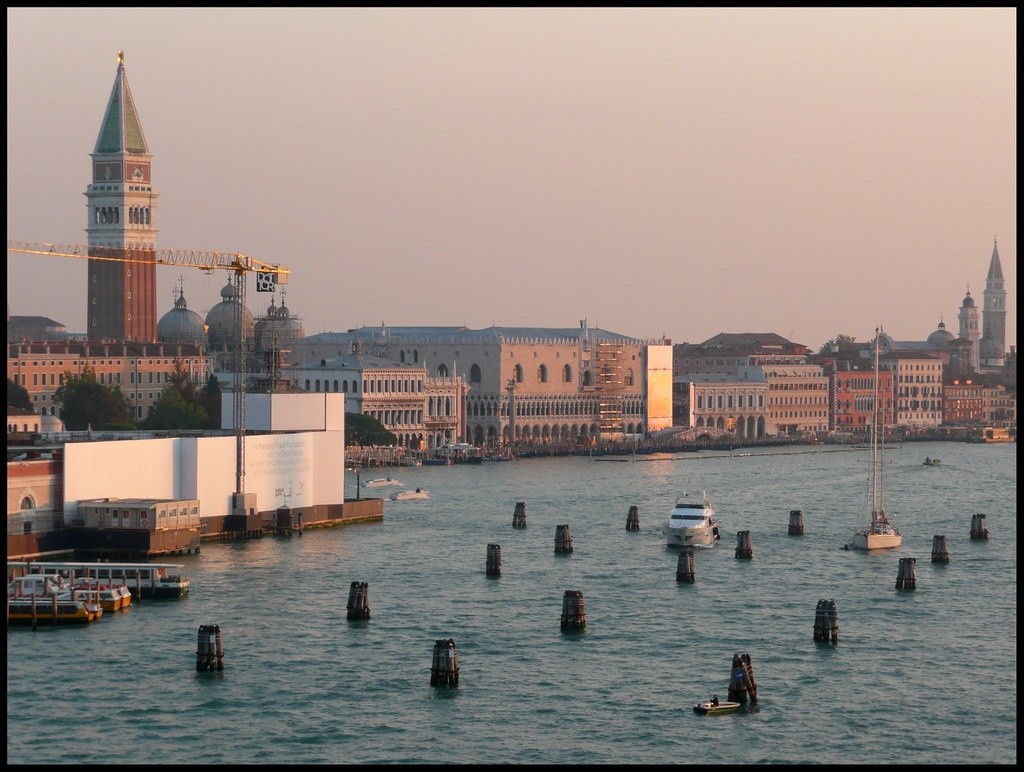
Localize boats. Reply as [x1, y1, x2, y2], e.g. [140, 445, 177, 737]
[359, 476, 402, 488]
[434, 442, 482, 464]
[423, 459, 451, 466]
[663, 480, 720, 551]
[922, 456, 941, 467]
[7, 561, 190, 622]
[389, 487, 431, 501]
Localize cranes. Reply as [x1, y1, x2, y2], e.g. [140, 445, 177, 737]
[7, 239, 290, 493]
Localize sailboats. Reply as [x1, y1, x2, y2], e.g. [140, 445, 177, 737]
[854, 327, 902, 549]
